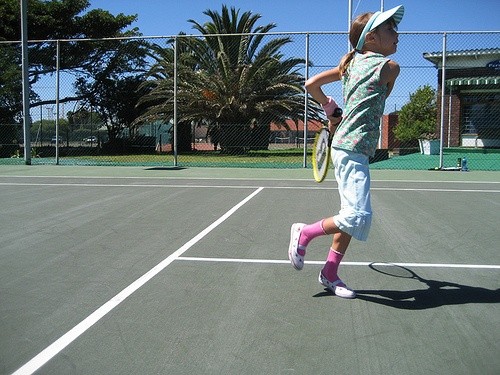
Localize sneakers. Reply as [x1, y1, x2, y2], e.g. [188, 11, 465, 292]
[288, 223, 304, 272]
[318, 271, 355, 299]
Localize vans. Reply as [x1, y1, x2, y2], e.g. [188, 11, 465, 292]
[52, 135, 63, 144]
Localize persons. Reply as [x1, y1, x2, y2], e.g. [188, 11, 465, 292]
[288, 4, 406, 299]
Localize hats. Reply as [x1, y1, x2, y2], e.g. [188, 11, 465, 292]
[356, 4, 405, 52]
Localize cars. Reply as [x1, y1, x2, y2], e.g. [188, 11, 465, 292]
[85, 136, 98, 143]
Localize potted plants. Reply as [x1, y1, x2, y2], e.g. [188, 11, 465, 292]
[391, 84, 440, 155]
